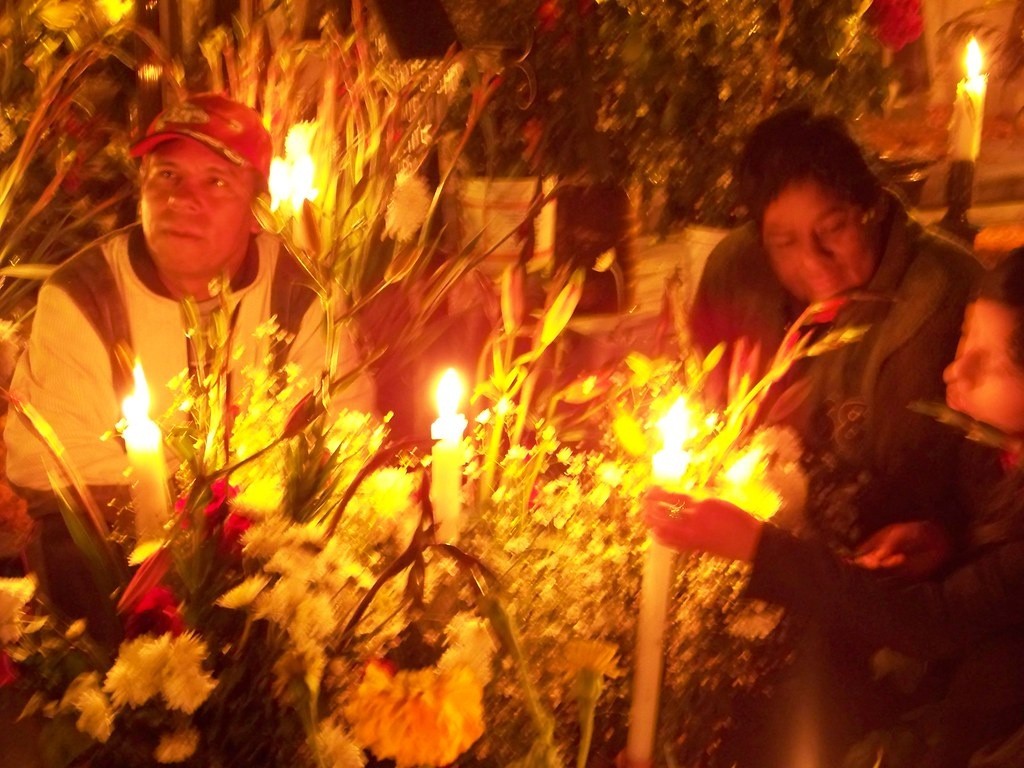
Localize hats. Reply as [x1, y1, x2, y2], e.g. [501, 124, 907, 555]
[127, 95, 272, 184]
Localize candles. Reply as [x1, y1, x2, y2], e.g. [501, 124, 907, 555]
[625, 397, 690, 768]
[119, 354, 169, 543]
[428, 369, 467, 548]
[946, 35, 988, 159]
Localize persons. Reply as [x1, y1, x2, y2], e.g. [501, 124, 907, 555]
[641, 246, 1024, 768]
[6, 92, 376, 521]
[686, 103, 1007, 560]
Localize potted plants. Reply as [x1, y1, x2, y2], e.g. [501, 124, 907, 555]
[597, 0, 887, 302]
[446, 45, 556, 276]
